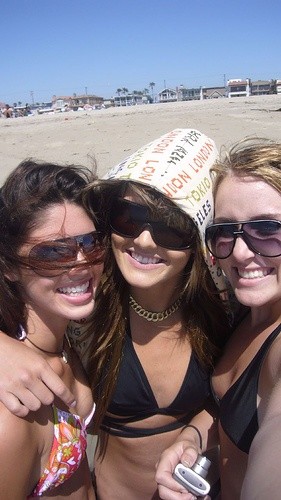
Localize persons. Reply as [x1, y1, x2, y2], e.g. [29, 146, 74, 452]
[0, 128, 230, 500]
[155, 137, 281, 500]
[0, 159, 105, 500]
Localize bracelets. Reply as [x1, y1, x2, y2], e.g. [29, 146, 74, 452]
[181, 424, 202, 449]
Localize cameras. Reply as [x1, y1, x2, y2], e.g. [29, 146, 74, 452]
[172, 454, 213, 497]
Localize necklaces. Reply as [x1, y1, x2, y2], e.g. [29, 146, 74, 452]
[26, 337, 68, 363]
[129, 295, 181, 322]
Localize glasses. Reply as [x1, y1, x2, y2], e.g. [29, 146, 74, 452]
[27, 230, 108, 279]
[203, 219, 280, 259]
[106, 198, 199, 252]
